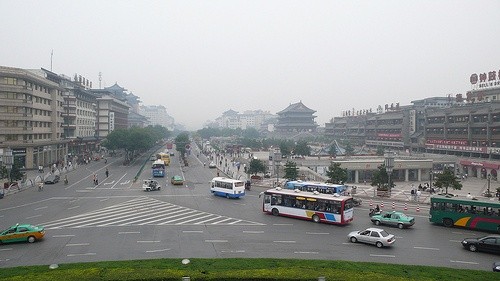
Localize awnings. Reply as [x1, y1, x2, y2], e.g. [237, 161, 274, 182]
[459, 161, 500, 169]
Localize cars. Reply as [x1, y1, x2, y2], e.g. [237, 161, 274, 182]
[43, 174, 60, 184]
[492, 262, 500, 272]
[348, 228, 396, 248]
[170, 175, 184, 185]
[209, 161, 216, 168]
[461, 235, 500, 256]
[371, 211, 415, 229]
[170, 152, 174, 156]
[165, 149, 169, 153]
[0, 223, 46, 246]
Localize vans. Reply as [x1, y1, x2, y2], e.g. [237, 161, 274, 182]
[151, 155, 156, 161]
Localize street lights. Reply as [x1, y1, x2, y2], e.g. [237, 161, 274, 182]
[58, 140, 73, 164]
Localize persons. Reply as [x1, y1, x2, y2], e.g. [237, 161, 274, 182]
[463, 173, 467, 180]
[458, 206, 500, 216]
[375, 204, 380, 212]
[220, 161, 222, 166]
[295, 187, 335, 194]
[272, 197, 341, 214]
[217, 169, 220, 176]
[466, 192, 472, 199]
[94, 175, 98, 185]
[418, 183, 443, 193]
[106, 169, 109, 177]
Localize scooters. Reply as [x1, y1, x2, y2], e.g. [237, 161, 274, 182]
[369, 208, 382, 216]
[245, 184, 251, 191]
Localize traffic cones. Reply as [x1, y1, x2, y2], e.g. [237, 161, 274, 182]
[416, 204, 421, 212]
[392, 202, 396, 209]
[369, 199, 373, 206]
[404, 203, 408, 210]
[380, 200, 384, 208]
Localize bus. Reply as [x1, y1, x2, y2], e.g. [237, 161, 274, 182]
[284, 180, 349, 196]
[208, 177, 245, 199]
[156, 152, 170, 166]
[151, 159, 165, 177]
[428, 197, 500, 235]
[167, 143, 172, 149]
[259, 187, 353, 225]
